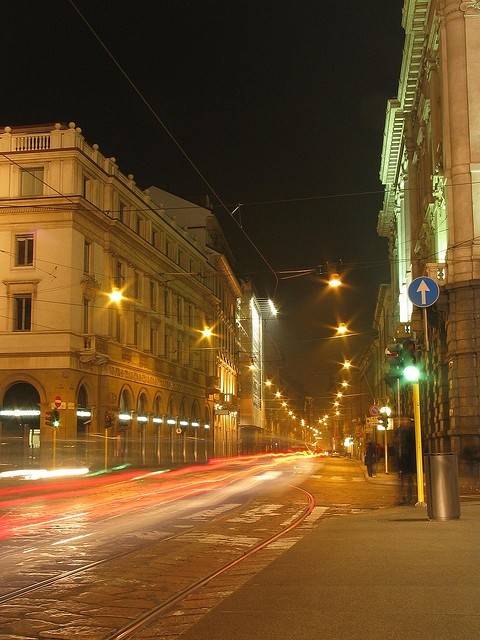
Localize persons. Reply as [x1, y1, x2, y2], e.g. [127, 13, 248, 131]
[364, 442, 376, 478]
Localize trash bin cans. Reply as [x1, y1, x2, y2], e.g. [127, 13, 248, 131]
[424, 452, 461, 522]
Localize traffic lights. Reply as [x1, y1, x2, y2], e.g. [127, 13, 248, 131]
[386, 340, 420, 382]
[45, 410, 60, 426]
[104, 412, 114, 427]
[378, 413, 388, 428]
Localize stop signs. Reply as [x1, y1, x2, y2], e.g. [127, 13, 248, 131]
[369, 404, 381, 417]
[54, 396, 61, 407]
